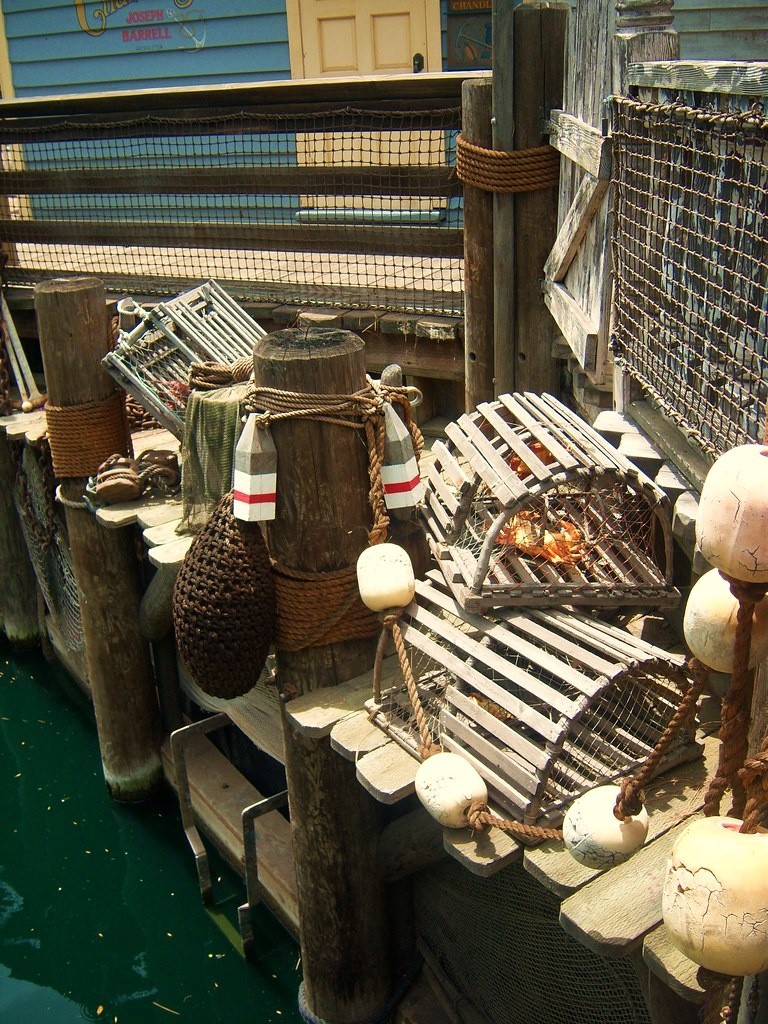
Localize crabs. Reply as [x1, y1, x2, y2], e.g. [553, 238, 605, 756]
[481, 441, 587, 564]
